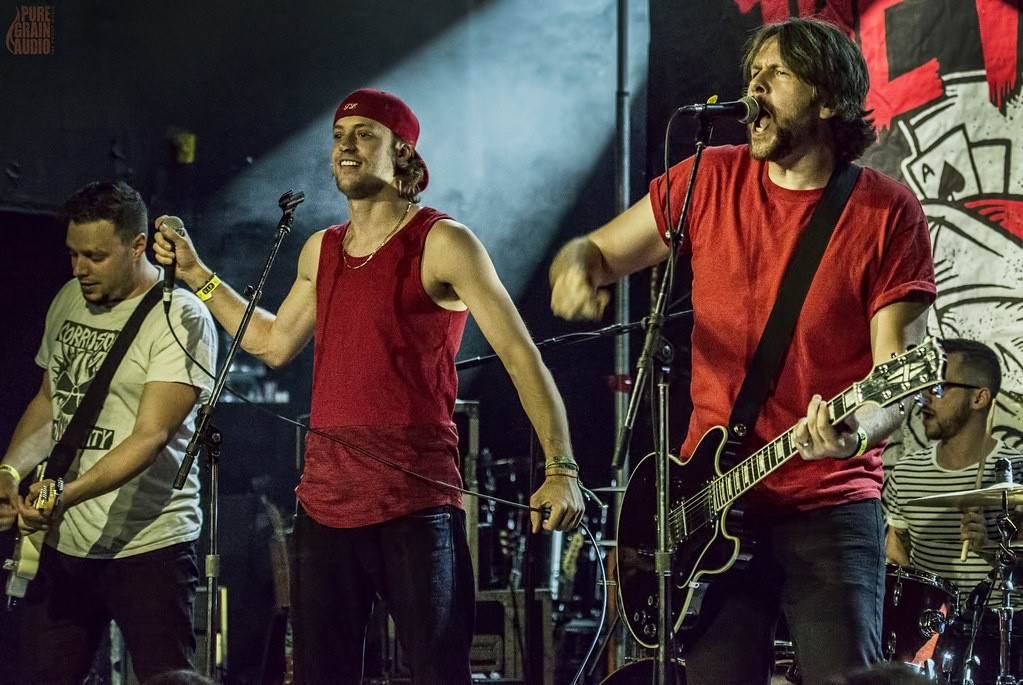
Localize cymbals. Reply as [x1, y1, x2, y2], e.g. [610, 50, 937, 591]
[907, 480, 1023, 507]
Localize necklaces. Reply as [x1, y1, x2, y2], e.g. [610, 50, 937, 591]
[339, 196, 411, 271]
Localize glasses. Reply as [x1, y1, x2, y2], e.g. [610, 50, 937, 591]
[942, 381, 981, 394]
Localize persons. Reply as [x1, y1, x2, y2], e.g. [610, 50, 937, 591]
[548, 19, 937, 685]
[149, 90, 585, 685]
[0, 182, 219, 685]
[884, 339, 1023, 647]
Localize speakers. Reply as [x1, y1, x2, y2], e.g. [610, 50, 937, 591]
[120, 585, 227, 685]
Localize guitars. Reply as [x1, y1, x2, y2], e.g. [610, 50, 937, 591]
[4, 461, 63, 598]
[614, 333, 949, 652]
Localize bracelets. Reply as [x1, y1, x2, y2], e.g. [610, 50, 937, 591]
[0, 464, 20, 484]
[834, 426, 868, 460]
[192, 273, 223, 301]
[544, 455, 578, 478]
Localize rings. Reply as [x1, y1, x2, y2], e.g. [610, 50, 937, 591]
[799, 435, 811, 449]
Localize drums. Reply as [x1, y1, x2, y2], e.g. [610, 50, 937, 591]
[597, 656, 688, 685]
[881, 563, 958, 677]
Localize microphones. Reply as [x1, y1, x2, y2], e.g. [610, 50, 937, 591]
[161, 216, 184, 313]
[678, 95, 761, 124]
[966, 568, 995, 611]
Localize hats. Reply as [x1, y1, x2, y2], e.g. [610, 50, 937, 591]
[333, 89, 420, 146]
